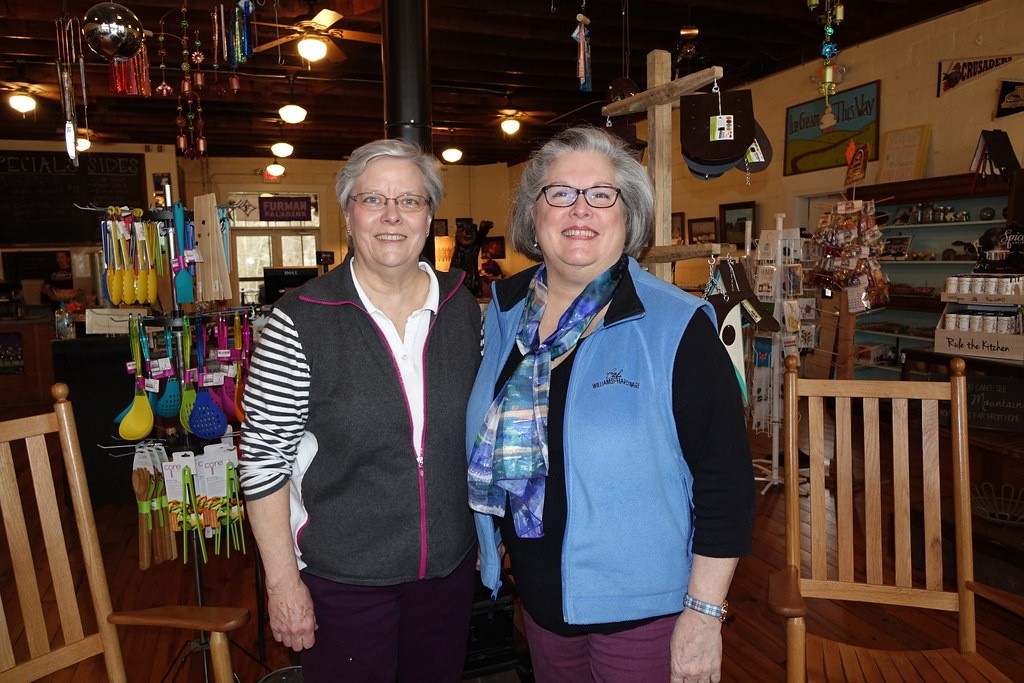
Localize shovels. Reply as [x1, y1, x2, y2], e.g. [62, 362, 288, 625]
[174, 201, 194, 304]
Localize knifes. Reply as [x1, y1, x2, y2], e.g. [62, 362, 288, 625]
[63, 71, 75, 159]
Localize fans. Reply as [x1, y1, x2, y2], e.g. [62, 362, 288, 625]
[77, 124, 126, 142]
[0, 62, 97, 106]
[250, 0, 382, 63]
[483, 91, 557, 127]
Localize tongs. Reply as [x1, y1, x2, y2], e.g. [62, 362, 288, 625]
[215, 526, 221, 555]
[230, 523, 240, 550]
[115, 206, 136, 305]
[182, 467, 209, 564]
[103, 209, 111, 302]
[107, 206, 124, 305]
[134, 208, 157, 304]
[226, 462, 246, 558]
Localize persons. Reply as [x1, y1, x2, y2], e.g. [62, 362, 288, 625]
[465, 129, 756, 683]
[236, 140, 482, 683]
[45, 251, 83, 314]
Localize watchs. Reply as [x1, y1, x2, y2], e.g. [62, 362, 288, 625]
[683, 594, 728, 623]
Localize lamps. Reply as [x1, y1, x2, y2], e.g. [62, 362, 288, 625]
[271, 120, 294, 157]
[500, 92, 522, 136]
[278, 76, 308, 124]
[75, 124, 91, 152]
[297, 0, 327, 62]
[266, 155, 285, 176]
[8, 62, 38, 113]
[442, 128, 463, 163]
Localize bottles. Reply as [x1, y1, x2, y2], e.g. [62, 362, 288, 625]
[0, 344, 24, 375]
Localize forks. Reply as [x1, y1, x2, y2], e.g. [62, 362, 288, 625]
[132, 465, 177, 570]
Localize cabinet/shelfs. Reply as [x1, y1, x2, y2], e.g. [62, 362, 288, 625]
[834, 169, 1024, 421]
[825, 410, 1024, 618]
[934, 273, 1024, 365]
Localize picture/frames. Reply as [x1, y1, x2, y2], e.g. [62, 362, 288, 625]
[783, 80, 881, 177]
[434, 219, 449, 236]
[719, 201, 757, 250]
[688, 217, 719, 258]
[672, 212, 685, 245]
[873, 123, 932, 185]
[481, 236, 506, 259]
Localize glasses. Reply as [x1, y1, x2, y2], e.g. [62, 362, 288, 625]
[348, 192, 430, 212]
[536, 185, 630, 212]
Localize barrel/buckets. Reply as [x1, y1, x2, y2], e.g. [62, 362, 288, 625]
[21, 280, 45, 306]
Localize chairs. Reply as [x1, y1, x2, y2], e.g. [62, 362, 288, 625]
[767, 356, 1024, 683]
[0, 383, 251, 683]
